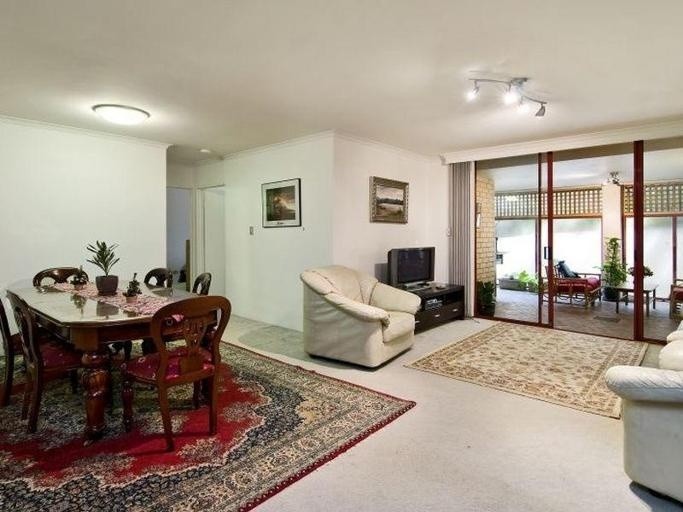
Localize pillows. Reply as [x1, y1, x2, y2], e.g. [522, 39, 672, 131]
[558, 260, 580, 279]
[659, 339, 683, 371]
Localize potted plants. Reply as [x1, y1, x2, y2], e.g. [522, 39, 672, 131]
[97, 301, 120, 320]
[70, 265, 88, 290]
[70, 293, 89, 314]
[86, 240, 120, 294]
[527, 272, 538, 292]
[518, 270, 530, 291]
[121, 273, 142, 303]
[591, 236, 629, 300]
[477, 280, 497, 317]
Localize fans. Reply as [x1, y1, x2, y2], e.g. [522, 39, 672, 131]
[602, 171, 623, 188]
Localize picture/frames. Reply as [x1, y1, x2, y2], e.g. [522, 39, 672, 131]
[544, 246, 547, 259]
[369, 176, 410, 224]
[262, 178, 303, 228]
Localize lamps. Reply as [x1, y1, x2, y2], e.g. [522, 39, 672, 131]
[91, 104, 151, 125]
[467, 77, 547, 116]
[475, 203, 481, 229]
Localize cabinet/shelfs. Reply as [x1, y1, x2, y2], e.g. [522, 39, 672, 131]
[394, 282, 465, 335]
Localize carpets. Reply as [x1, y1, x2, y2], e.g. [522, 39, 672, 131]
[402, 322, 650, 420]
[0, 338, 417, 512]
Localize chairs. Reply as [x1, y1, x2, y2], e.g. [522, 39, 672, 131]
[141, 272, 211, 356]
[541, 264, 601, 310]
[33, 266, 89, 288]
[123, 268, 172, 356]
[668, 279, 683, 319]
[1, 299, 49, 405]
[6, 290, 114, 433]
[118, 295, 231, 451]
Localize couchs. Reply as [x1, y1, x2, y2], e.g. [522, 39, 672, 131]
[300, 265, 420, 372]
[604, 329, 683, 504]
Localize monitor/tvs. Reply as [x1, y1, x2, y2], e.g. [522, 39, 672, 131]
[387, 246, 435, 287]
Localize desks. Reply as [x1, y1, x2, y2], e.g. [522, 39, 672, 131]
[5, 282, 217, 439]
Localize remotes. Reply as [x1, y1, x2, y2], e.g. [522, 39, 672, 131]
[436, 284, 446, 289]
